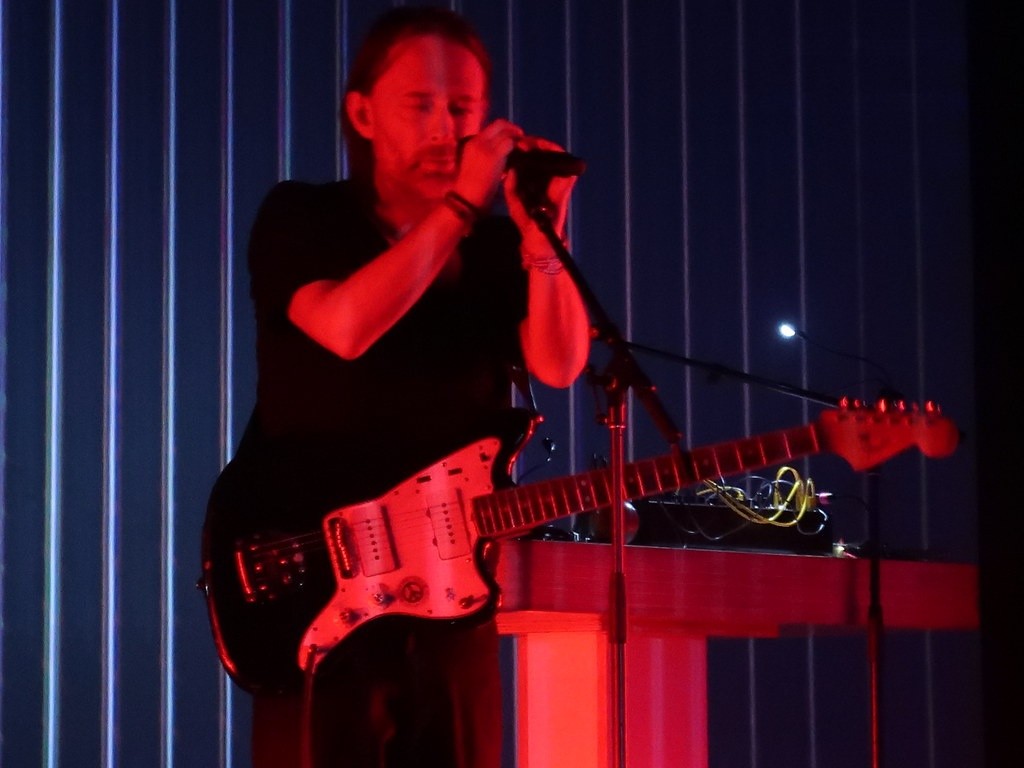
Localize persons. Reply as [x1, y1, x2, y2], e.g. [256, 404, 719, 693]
[248, 3, 590, 768]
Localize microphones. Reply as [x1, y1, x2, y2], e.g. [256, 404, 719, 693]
[455, 135, 586, 179]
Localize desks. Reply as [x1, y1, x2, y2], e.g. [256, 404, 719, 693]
[483, 539, 979, 768]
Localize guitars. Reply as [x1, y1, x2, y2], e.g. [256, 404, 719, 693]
[202, 394, 960, 684]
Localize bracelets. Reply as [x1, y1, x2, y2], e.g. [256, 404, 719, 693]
[534, 260, 564, 275]
